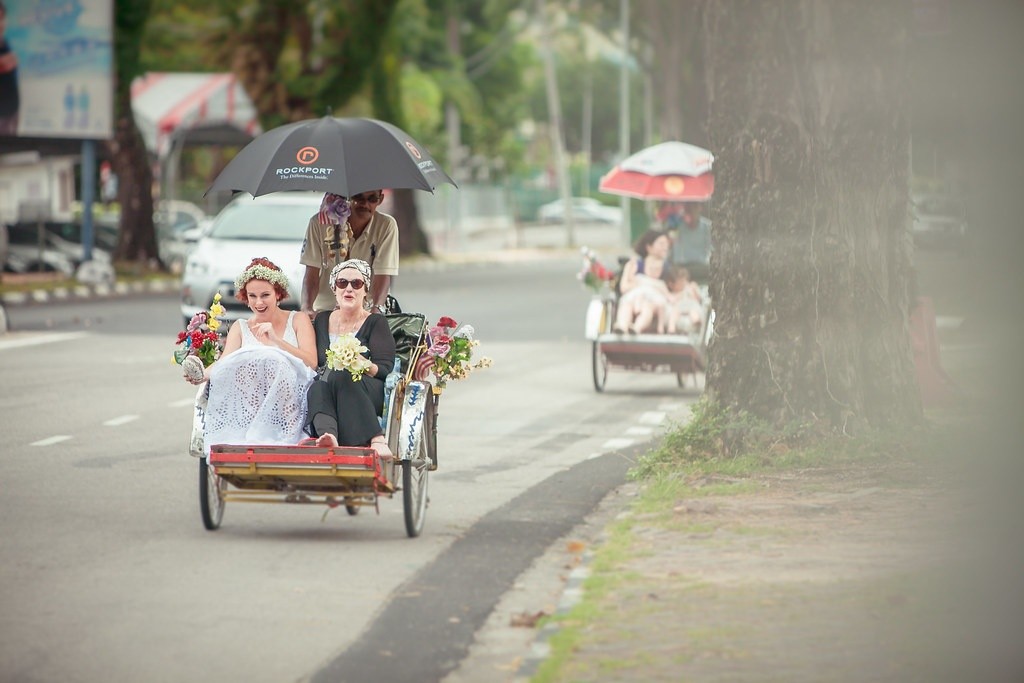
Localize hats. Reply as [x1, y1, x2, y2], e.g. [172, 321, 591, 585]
[328, 258, 371, 294]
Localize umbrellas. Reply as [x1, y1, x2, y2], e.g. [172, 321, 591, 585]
[202, 105, 459, 200]
[599, 141, 714, 202]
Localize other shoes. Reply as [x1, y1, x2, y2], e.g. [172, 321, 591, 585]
[371, 438, 393, 460]
[315, 432, 339, 447]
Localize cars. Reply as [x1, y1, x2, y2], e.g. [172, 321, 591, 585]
[173, 189, 333, 332]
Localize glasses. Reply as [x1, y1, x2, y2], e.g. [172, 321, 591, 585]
[335, 278, 364, 290]
[350, 194, 381, 203]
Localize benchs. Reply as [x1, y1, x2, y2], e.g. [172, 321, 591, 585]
[617, 258, 710, 297]
[311, 312, 430, 381]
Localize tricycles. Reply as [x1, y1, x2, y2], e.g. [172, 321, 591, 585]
[587, 239, 714, 392]
[187, 300, 440, 538]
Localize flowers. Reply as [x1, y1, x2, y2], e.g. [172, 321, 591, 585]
[423, 315, 492, 387]
[578, 250, 615, 296]
[320, 333, 373, 383]
[171, 292, 226, 367]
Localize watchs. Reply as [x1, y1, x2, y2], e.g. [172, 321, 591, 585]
[373, 304, 385, 313]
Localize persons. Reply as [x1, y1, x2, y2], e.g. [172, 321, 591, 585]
[182, 256, 318, 464]
[298, 189, 400, 320]
[302, 259, 396, 459]
[670, 201, 712, 264]
[613, 230, 702, 336]
